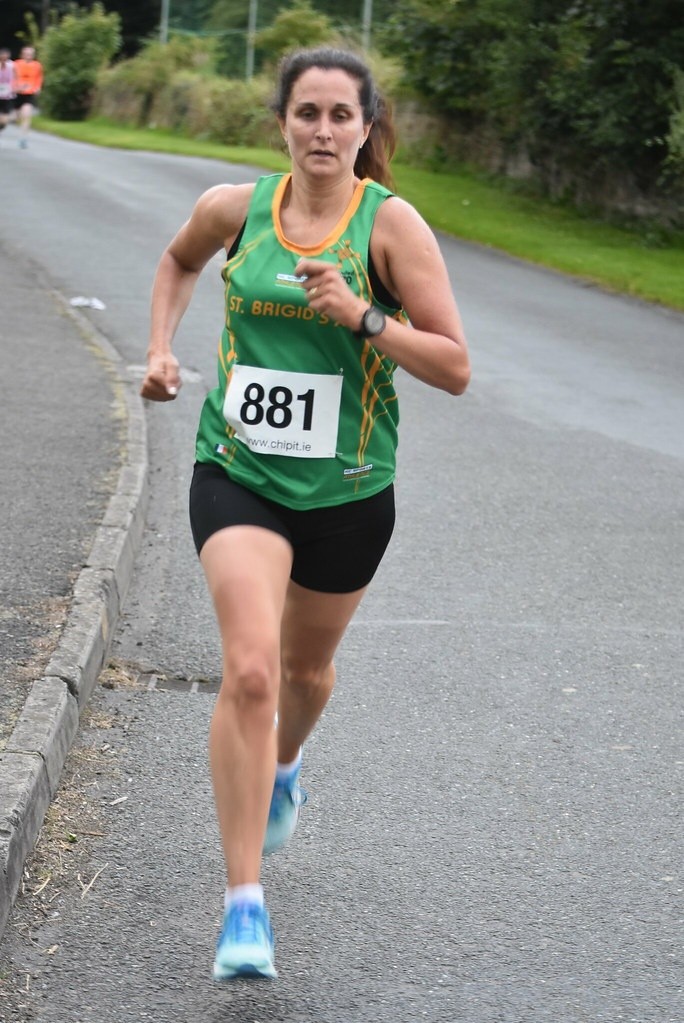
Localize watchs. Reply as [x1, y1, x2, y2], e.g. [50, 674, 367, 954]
[352, 304, 386, 341]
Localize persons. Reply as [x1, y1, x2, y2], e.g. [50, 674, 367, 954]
[0, 49, 17, 131]
[139, 51, 469, 984]
[11, 46, 44, 149]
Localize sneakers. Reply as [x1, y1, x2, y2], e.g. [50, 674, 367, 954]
[211, 903, 277, 979]
[263, 764, 302, 856]
